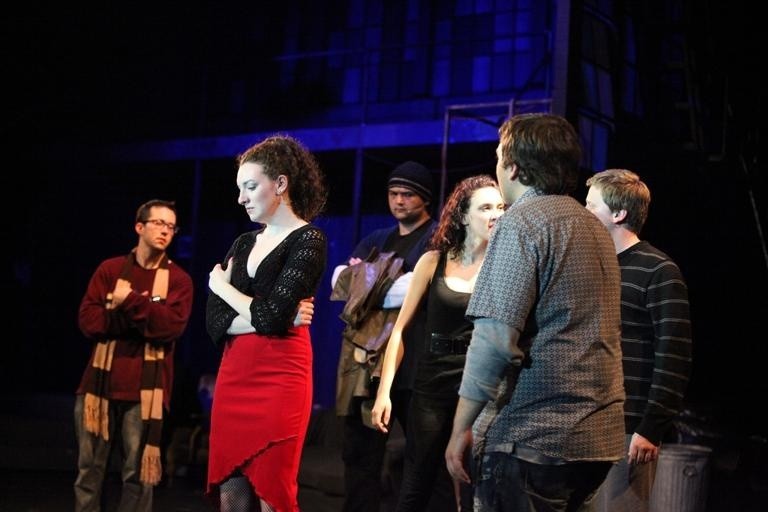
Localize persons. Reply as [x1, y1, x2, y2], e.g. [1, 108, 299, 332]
[70, 199, 195, 511]
[366, 174, 506, 511]
[442, 112, 629, 511]
[200, 132, 329, 511]
[580, 167, 721, 511]
[328, 160, 444, 512]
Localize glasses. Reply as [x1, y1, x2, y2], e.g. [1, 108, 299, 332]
[144, 219, 179, 233]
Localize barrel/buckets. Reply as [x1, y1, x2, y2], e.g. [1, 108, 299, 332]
[648, 444, 712, 512]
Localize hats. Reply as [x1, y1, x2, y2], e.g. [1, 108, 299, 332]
[388, 161, 432, 202]
